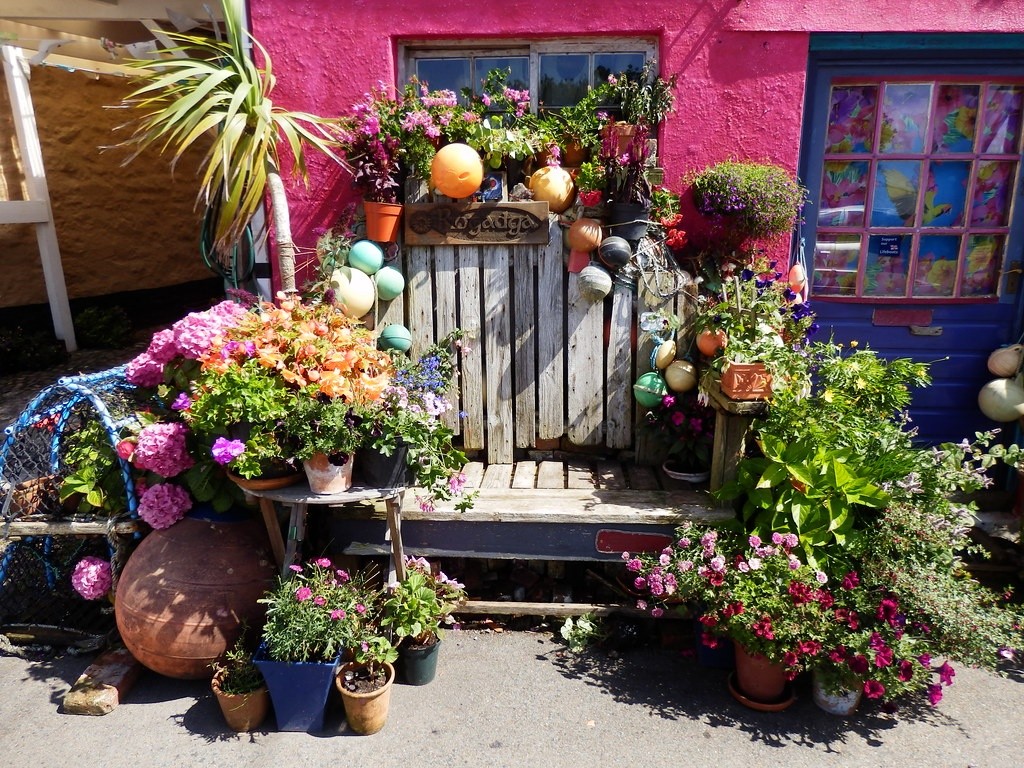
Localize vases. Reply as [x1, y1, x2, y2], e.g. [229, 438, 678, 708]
[362, 201, 401, 244]
[212, 667, 269, 731]
[393, 632, 441, 686]
[335, 659, 396, 735]
[253, 641, 343, 731]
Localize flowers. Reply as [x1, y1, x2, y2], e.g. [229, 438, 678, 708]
[550, 152, 1023, 716]
[327, 76, 669, 205]
[34, 286, 474, 667]
